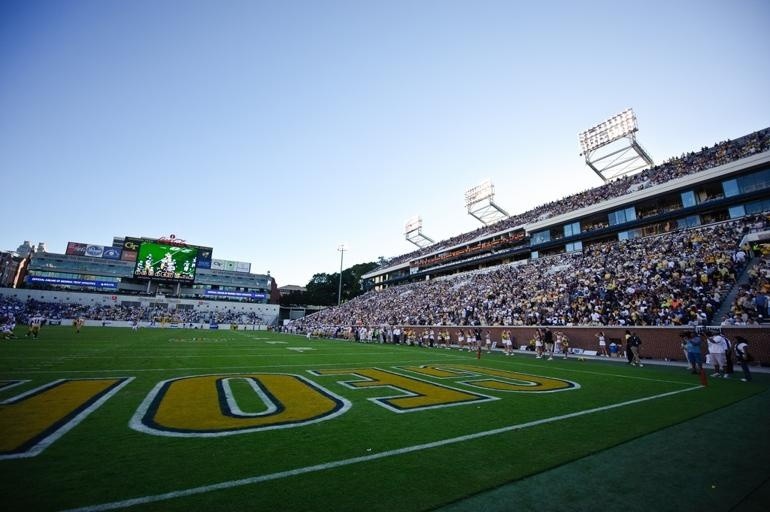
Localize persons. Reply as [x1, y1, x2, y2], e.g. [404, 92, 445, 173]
[2, 281, 271, 340]
[271, 128, 770, 380]
[136, 252, 197, 279]
